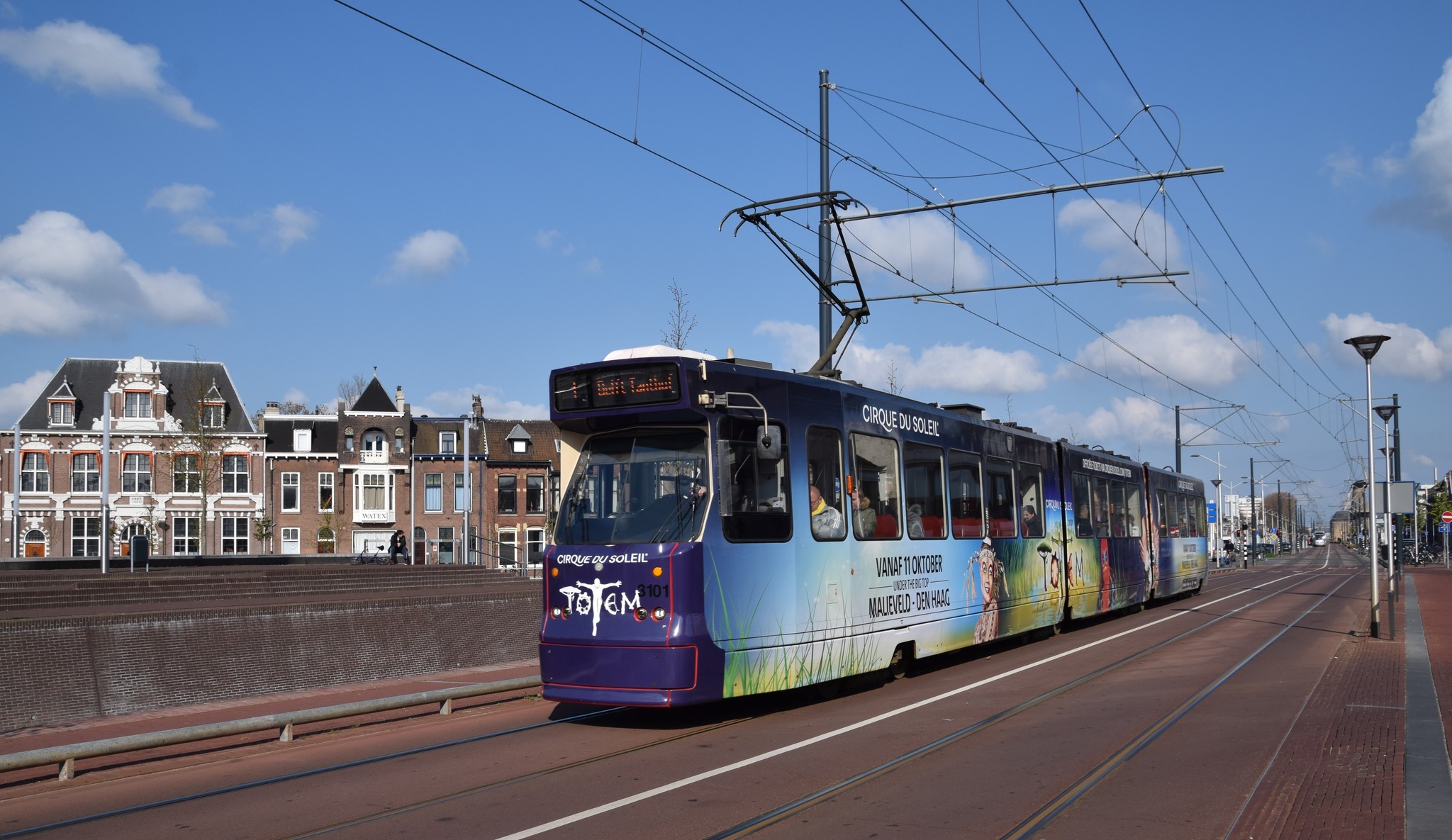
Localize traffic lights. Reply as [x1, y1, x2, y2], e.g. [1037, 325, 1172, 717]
[1235, 529, 1241, 539]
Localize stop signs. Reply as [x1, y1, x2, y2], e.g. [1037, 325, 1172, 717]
[1441, 511, 1452, 523]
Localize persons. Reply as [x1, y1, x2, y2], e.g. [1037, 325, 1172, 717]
[810, 485, 844, 538]
[1023, 506, 1043, 536]
[1075, 488, 1140, 537]
[852, 485, 877, 538]
[690, 464, 744, 512]
[1223, 539, 1235, 561]
[1310, 536, 1313, 546]
[1159, 508, 1196, 536]
[389, 529, 410, 565]
[894, 500, 924, 537]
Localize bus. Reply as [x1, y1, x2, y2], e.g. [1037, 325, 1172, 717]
[539, 190, 1208, 710]
[1313, 523, 1327, 546]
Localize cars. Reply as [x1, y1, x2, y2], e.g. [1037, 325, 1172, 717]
[1212, 546, 1236, 562]
[1246, 542, 1291, 558]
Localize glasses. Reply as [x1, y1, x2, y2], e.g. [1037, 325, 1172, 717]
[811, 497, 820, 506]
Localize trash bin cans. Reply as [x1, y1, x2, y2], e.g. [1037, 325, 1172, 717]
[129, 537, 148, 564]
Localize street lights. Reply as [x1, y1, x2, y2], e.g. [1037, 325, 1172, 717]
[1209, 479, 1224, 569]
[1342, 334, 1393, 639]
[1371, 405, 1402, 591]
[1241, 475, 1269, 560]
[1238, 495, 1259, 558]
[1245, 500, 1289, 544]
[1221, 481, 1250, 545]
[411, 437, 417, 566]
[1190, 451, 1227, 566]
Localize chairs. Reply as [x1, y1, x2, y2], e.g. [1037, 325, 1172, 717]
[977, 516, 1000, 537]
[939, 517, 965, 539]
[874, 514, 898, 537]
[1112, 523, 1123, 537]
[996, 519, 1015, 537]
[920, 516, 943, 538]
[960, 517, 983, 537]
[1011, 518, 1031, 536]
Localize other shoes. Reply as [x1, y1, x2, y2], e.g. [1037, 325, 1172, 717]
[1228, 558, 1231, 561]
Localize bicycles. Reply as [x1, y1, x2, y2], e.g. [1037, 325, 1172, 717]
[1402, 542, 1452, 566]
[1360, 545, 1381, 557]
[1221, 547, 1233, 568]
[350, 545, 390, 565]
